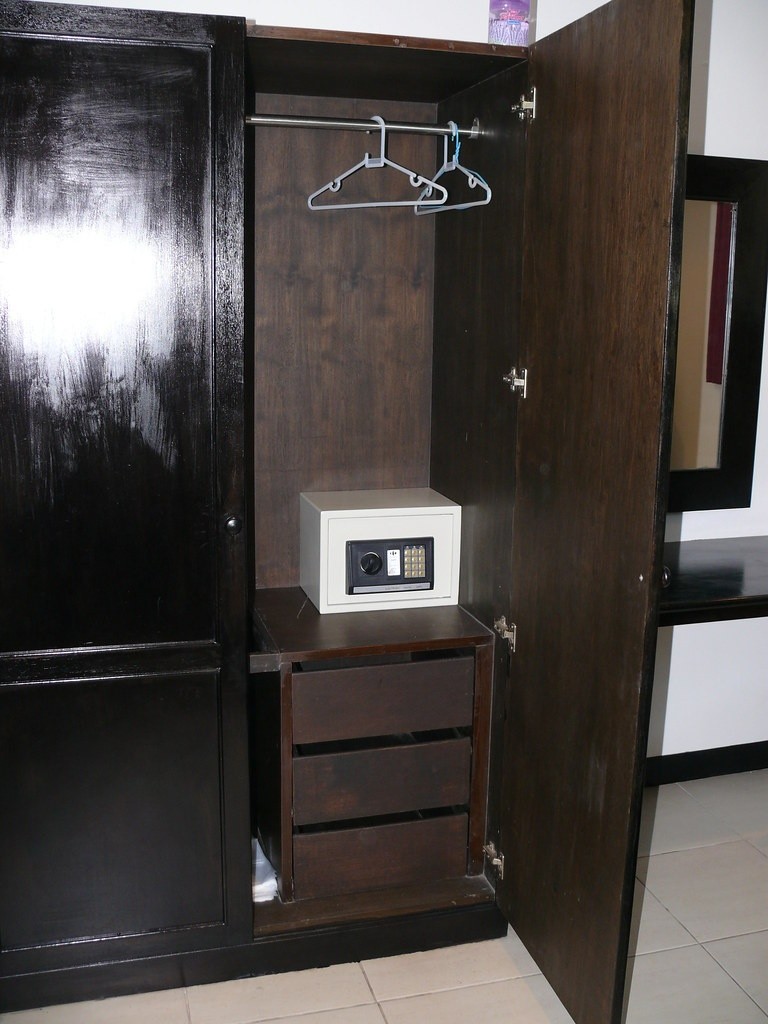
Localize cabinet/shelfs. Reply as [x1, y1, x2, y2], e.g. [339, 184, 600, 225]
[0, 0, 695, 1024]
[644, 535, 768, 787]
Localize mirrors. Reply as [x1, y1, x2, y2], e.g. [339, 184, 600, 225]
[666, 153, 768, 512]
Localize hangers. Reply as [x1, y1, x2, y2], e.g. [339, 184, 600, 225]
[307, 115, 446, 210]
[419, 124, 488, 208]
[414, 121, 491, 215]
